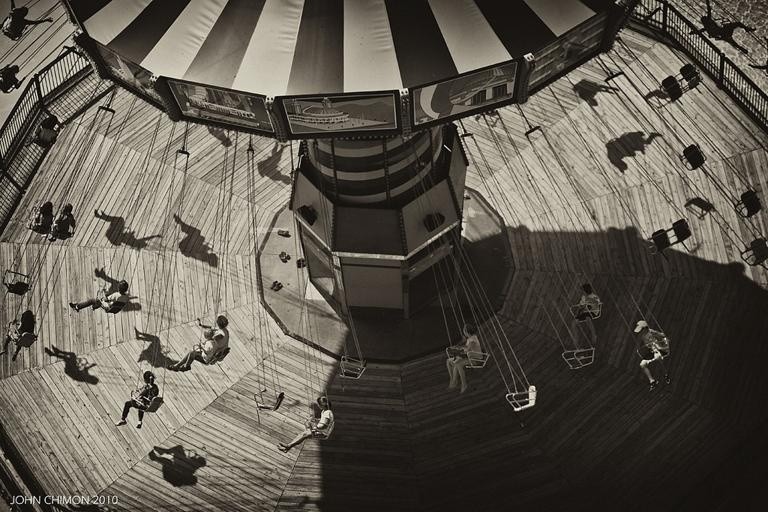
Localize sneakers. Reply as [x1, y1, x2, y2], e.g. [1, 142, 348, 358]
[69, 302, 80, 313]
[277, 441, 291, 453]
[116, 420, 126, 426]
[0, 350, 6, 356]
[12, 352, 17, 362]
[648, 380, 658, 392]
[136, 421, 142, 428]
[167, 364, 191, 372]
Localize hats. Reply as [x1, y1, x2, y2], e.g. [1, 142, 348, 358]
[634, 320, 648, 333]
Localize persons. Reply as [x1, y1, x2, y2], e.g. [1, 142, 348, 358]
[444, 323, 484, 395]
[571, 283, 600, 349]
[0, 0, 54, 41]
[114, 370, 158, 430]
[69, 280, 130, 314]
[634, 320, 672, 392]
[1, 63, 27, 92]
[25, 202, 77, 241]
[168, 314, 230, 372]
[0, 309, 35, 361]
[33, 113, 59, 148]
[277, 395, 335, 455]
